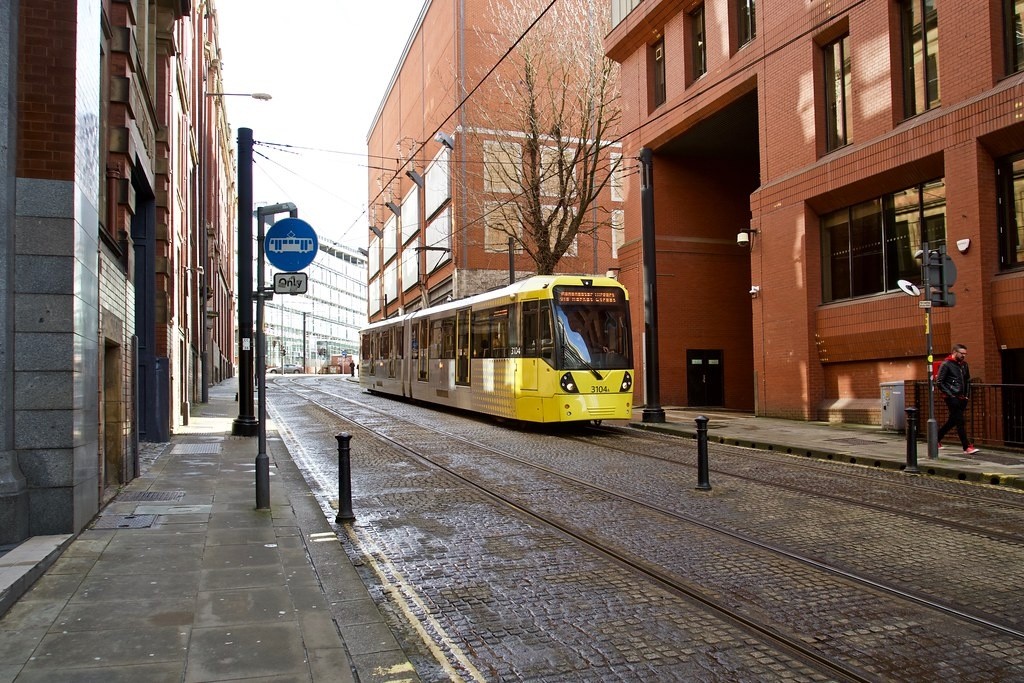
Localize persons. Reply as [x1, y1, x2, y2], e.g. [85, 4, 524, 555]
[349, 360, 355, 376]
[936, 345, 980, 455]
[570, 319, 608, 357]
[479, 331, 502, 358]
[356, 362, 359, 377]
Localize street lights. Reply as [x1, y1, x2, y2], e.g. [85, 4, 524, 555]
[202, 92, 274, 405]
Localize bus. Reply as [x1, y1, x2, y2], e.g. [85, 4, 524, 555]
[359, 274, 635, 435]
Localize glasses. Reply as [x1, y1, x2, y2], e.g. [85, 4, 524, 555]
[956, 350, 969, 355]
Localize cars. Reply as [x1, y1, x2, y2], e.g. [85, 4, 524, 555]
[266, 364, 303, 375]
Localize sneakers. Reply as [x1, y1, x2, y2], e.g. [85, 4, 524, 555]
[938, 442, 945, 450]
[963, 444, 980, 454]
